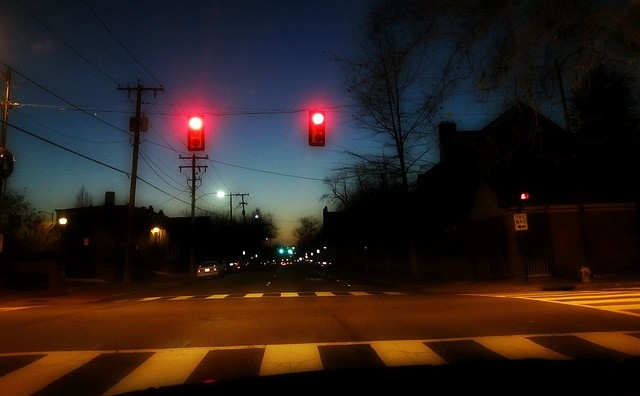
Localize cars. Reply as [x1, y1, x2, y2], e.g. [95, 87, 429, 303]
[196, 260, 226, 280]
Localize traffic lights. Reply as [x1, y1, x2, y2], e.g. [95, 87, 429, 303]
[187, 114, 205, 151]
[519, 190, 530, 202]
[309, 108, 325, 145]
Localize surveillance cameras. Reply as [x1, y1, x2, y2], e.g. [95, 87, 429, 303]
[519, 192, 531, 201]
